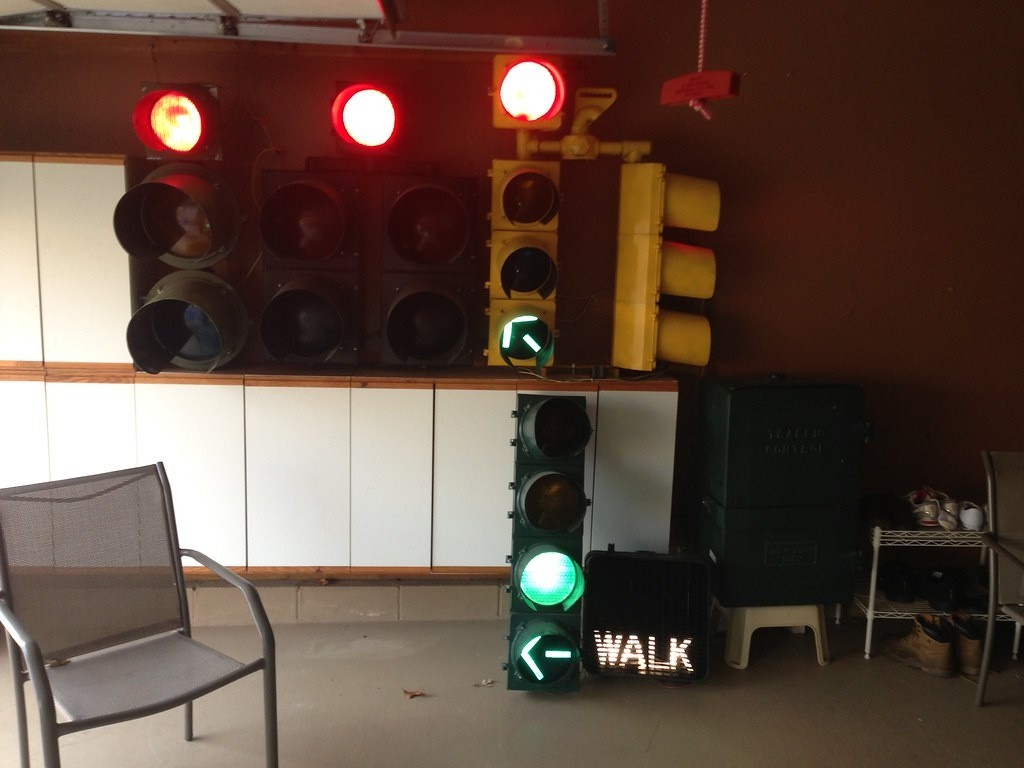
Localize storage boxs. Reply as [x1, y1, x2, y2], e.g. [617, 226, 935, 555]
[681, 475, 864, 609]
[688, 377, 874, 509]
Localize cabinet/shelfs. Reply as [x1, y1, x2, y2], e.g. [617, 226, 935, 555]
[1, 151, 682, 581]
[836, 509, 1022, 661]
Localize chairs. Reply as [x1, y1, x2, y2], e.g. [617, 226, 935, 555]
[0, 460, 279, 767]
[974, 448, 1024, 710]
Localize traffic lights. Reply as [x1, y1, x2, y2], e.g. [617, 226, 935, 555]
[478, 157, 559, 373]
[577, 543, 713, 686]
[610, 157, 722, 375]
[489, 50, 567, 133]
[501, 389, 594, 694]
[111, 76, 490, 372]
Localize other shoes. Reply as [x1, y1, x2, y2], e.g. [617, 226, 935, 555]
[889, 564, 960, 610]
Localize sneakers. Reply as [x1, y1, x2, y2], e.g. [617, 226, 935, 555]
[866, 486, 984, 532]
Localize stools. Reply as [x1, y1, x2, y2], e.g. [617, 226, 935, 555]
[710, 595, 833, 670]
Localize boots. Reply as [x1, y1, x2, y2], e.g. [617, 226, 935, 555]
[945, 612, 983, 674]
[876, 612, 955, 678]
